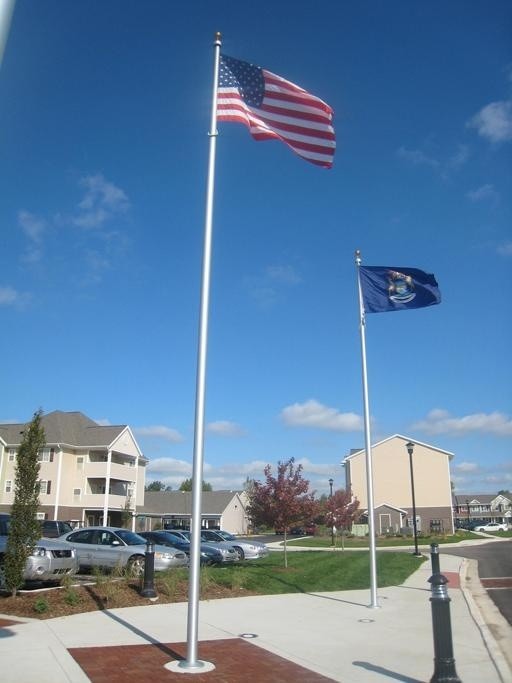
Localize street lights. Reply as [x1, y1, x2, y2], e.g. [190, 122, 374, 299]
[328, 478, 335, 546]
[405, 440, 422, 556]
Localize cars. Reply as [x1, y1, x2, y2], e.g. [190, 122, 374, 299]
[158, 530, 240, 562]
[135, 531, 224, 567]
[474, 522, 509, 532]
[212, 530, 270, 561]
[58, 525, 188, 578]
[0, 512, 80, 590]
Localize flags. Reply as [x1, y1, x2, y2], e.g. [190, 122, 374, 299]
[358, 264, 443, 315]
[213, 29, 336, 171]
